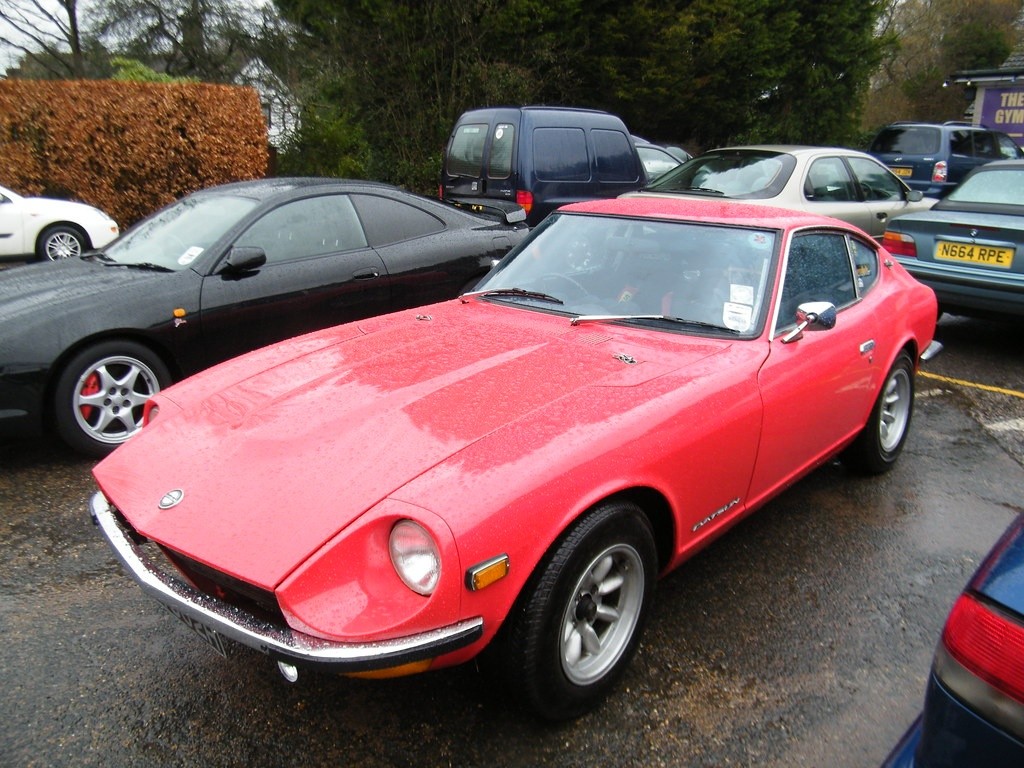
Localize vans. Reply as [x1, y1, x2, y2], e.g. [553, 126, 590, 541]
[438, 106, 684, 228]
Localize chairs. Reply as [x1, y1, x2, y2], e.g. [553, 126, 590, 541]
[663, 237, 762, 335]
[596, 234, 682, 320]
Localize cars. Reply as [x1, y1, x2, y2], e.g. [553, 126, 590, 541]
[882, 160, 1024, 322]
[879, 510, 1024, 768]
[90, 198, 942, 724]
[0, 184, 119, 262]
[0, 177, 530, 459]
[616, 145, 939, 244]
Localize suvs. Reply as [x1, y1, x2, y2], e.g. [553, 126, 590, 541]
[862, 121, 1024, 201]
